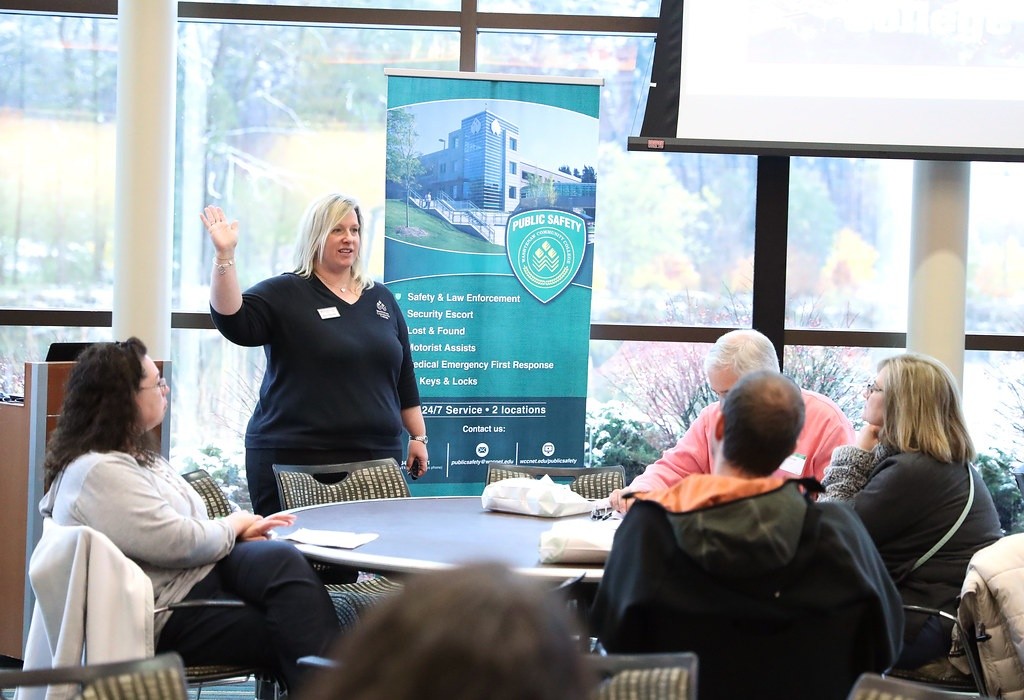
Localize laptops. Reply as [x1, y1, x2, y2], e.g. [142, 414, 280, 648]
[8, 341, 115, 403]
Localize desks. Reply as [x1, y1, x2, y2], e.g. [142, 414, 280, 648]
[240, 495, 639, 584]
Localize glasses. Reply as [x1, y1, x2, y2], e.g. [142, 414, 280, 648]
[865, 384, 882, 394]
[138, 378, 167, 390]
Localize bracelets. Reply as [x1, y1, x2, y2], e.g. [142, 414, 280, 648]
[212, 257, 236, 275]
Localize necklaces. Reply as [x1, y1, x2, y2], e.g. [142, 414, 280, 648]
[316, 271, 354, 292]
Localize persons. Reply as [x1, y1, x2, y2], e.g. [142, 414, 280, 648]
[200, 193, 431, 516]
[611, 330, 855, 515]
[36, 338, 351, 700]
[308, 560, 598, 700]
[811, 352, 1006, 666]
[590, 370, 907, 700]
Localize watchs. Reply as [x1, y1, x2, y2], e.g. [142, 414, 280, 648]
[408, 435, 429, 444]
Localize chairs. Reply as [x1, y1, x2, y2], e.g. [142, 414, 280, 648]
[278, 457, 429, 618]
[485, 455, 634, 508]
